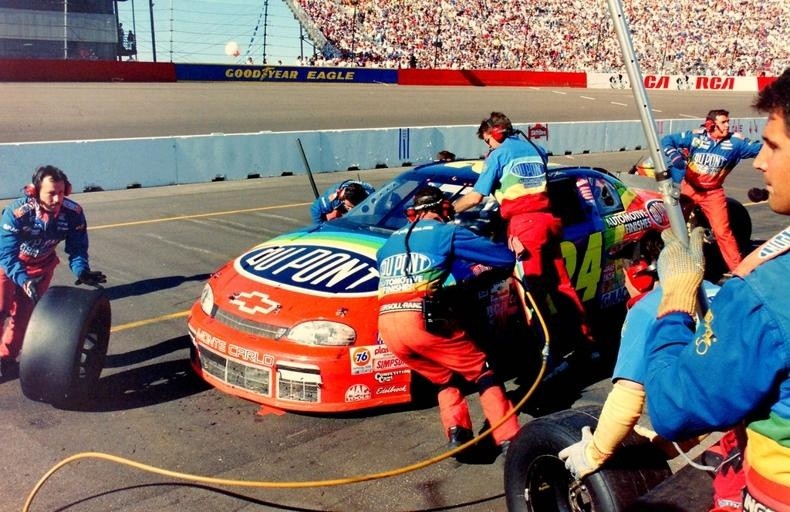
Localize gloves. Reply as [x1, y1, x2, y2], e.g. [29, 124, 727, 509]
[558, 427, 600, 481]
[22, 275, 48, 301]
[670, 151, 686, 170]
[74, 269, 108, 289]
[657, 227, 707, 321]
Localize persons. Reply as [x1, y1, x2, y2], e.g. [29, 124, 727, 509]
[450, 112, 605, 374]
[643, 62, 790, 511]
[247, 57, 255, 65]
[0, 163, 109, 386]
[1, 2, 136, 61]
[437, 150, 455, 161]
[277, 60, 285, 66]
[295, 1, 790, 77]
[308, 177, 376, 224]
[558, 226, 743, 486]
[375, 187, 528, 465]
[660, 107, 762, 275]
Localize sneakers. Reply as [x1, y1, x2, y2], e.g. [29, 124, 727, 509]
[449, 427, 477, 457]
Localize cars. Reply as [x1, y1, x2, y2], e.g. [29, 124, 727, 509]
[188, 159, 714, 413]
